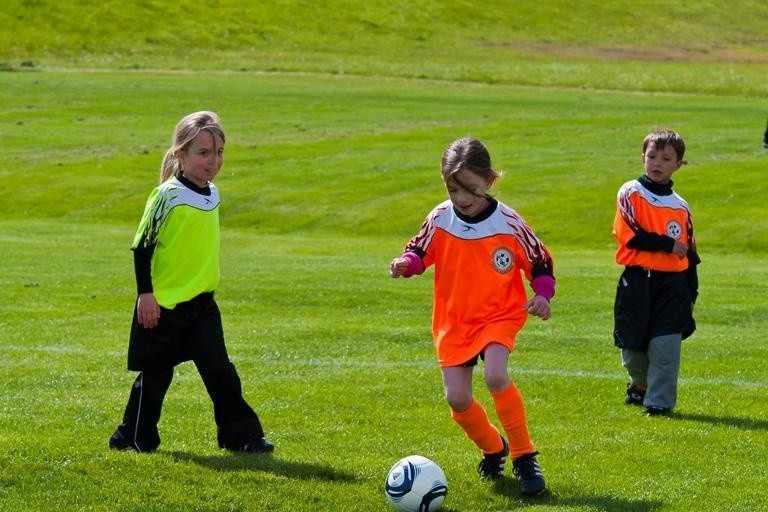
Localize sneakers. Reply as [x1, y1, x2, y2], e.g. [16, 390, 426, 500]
[478, 434, 509, 481]
[640, 405, 673, 416]
[511, 450, 549, 496]
[622, 382, 648, 406]
[217, 437, 275, 452]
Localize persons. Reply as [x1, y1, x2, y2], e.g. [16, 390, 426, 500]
[391, 138, 556, 495]
[613, 128, 702, 418]
[109, 111, 275, 454]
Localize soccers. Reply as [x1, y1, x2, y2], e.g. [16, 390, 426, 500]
[383, 455, 447, 512]
